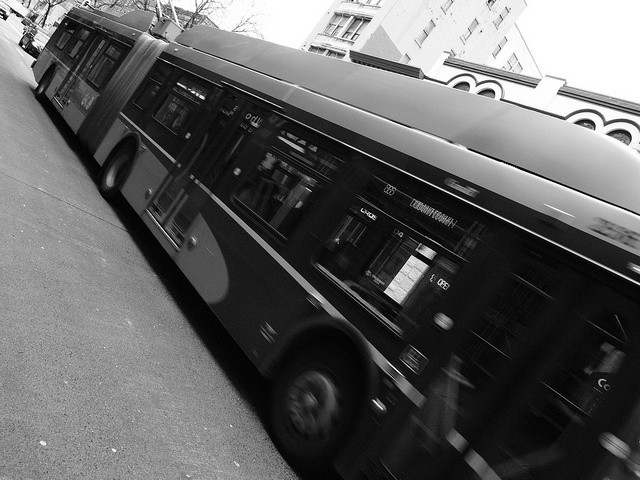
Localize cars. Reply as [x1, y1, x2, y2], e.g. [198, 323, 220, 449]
[19, 29, 50, 58]
[0, 1, 12, 21]
[23, 24, 47, 36]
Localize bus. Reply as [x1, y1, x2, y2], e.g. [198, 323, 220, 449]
[31, 8, 640, 479]
